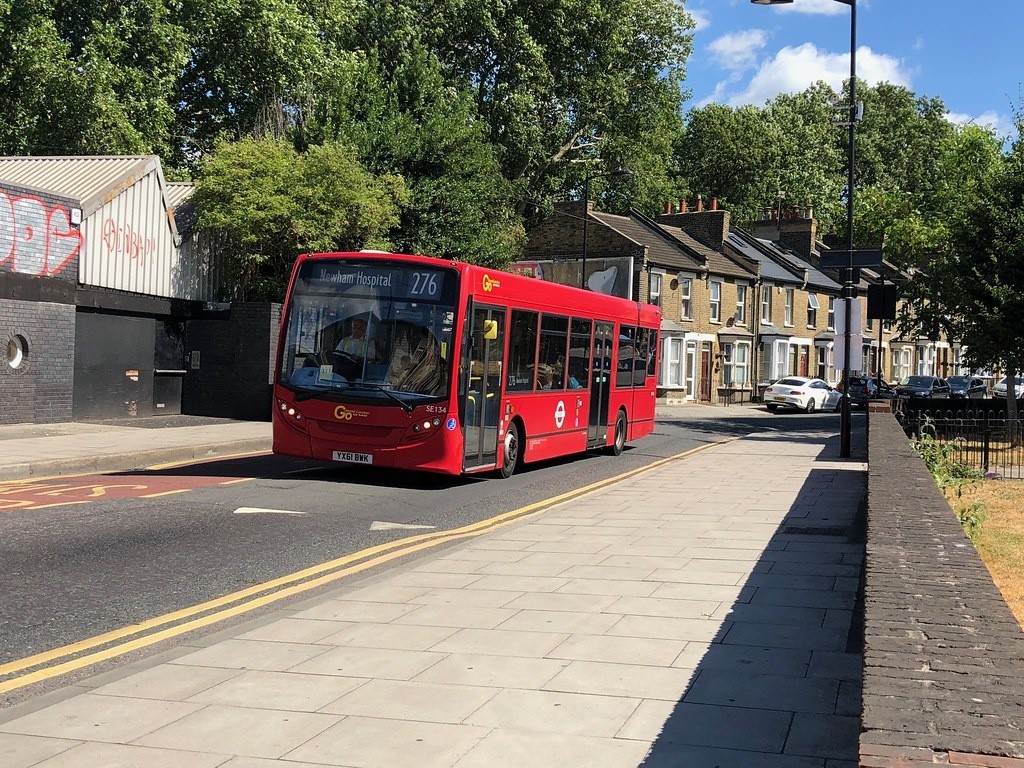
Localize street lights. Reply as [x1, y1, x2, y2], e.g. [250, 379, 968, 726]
[750, 0, 859, 458]
[575, 169, 634, 290]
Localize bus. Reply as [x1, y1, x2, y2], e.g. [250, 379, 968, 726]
[271, 248, 663, 482]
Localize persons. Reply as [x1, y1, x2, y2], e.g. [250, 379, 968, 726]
[869, 368, 884, 380]
[335, 319, 375, 359]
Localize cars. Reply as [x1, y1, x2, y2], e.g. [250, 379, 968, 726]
[991, 374, 1024, 400]
[834, 375, 897, 404]
[943, 374, 988, 399]
[891, 373, 952, 402]
[762, 376, 852, 415]
